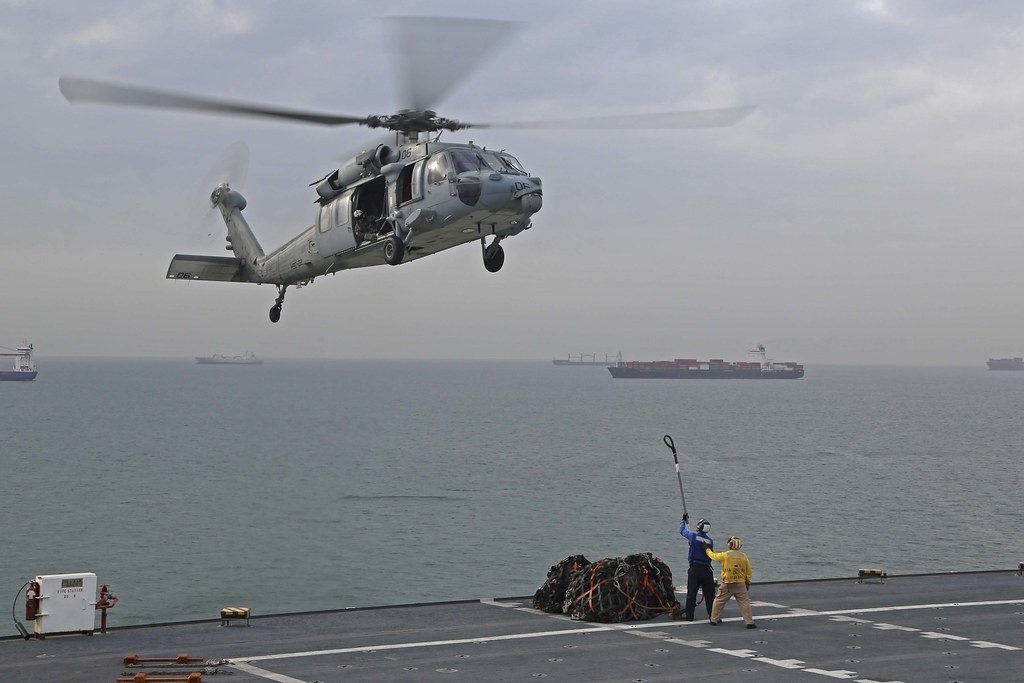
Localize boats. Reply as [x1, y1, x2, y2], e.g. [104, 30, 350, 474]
[608, 345, 805, 381]
[0, 346, 37, 381]
[199, 349, 261, 366]
[987, 358, 1024, 371]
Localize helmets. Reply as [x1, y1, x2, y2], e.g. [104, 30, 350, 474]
[353, 209, 364, 218]
[697, 519, 712, 533]
[728, 536, 743, 551]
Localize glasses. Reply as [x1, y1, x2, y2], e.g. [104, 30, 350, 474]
[355, 215, 364, 220]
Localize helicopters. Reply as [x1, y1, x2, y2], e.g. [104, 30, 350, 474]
[58, 13, 754, 324]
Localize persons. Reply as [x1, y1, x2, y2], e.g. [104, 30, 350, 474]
[679, 513, 722, 623]
[353, 210, 377, 238]
[699, 536, 757, 629]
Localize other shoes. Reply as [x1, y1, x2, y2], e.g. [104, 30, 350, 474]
[686, 614, 694, 621]
[710, 622, 717, 626]
[747, 624, 757, 629]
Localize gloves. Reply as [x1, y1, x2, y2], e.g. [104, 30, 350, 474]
[699, 541, 709, 549]
[683, 512, 690, 520]
